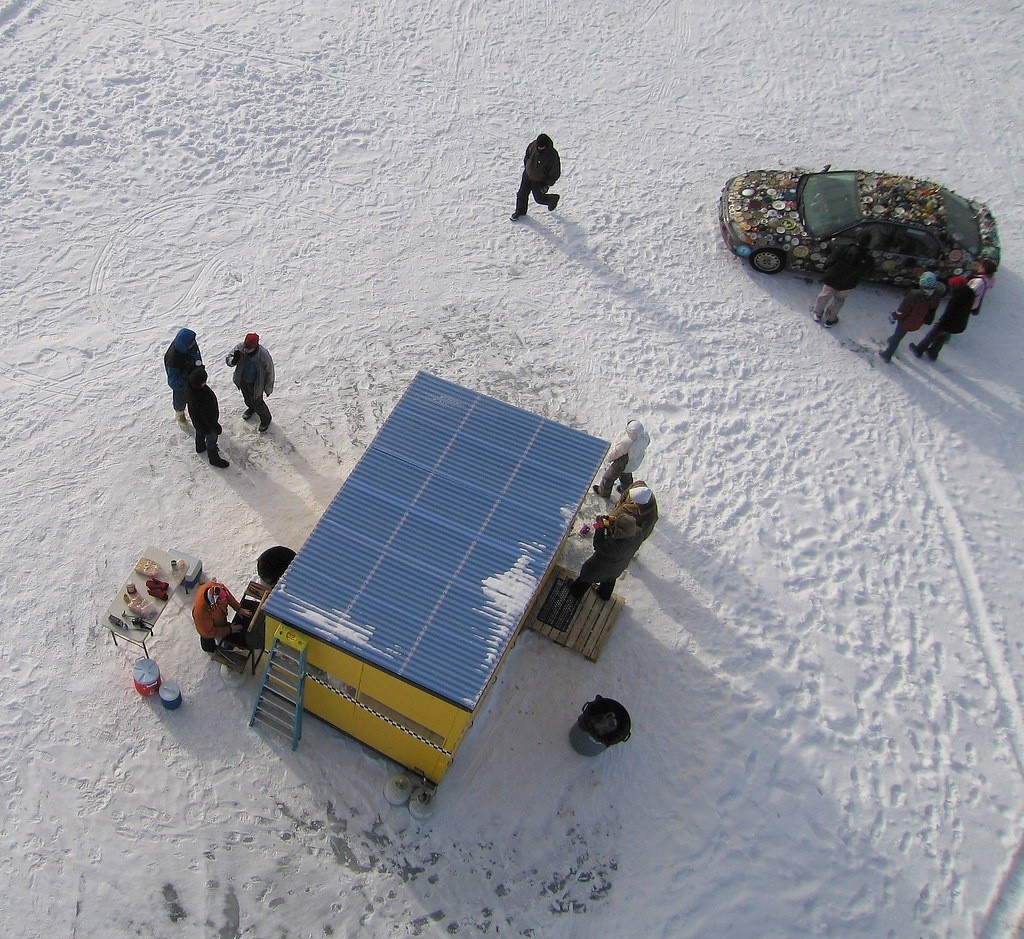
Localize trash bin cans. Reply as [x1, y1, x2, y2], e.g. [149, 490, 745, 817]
[258, 546, 296, 587]
[569, 694, 632, 759]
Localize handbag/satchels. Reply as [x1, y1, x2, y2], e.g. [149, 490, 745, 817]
[971, 308, 980, 316]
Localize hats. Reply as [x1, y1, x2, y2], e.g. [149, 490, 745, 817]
[245, 334, 259, 345]
[919, 272, 936, 287]
[189, 368, 207, 385]
[629, 488, 652, 504]
[537, 135, 546, 147]
[948, 277, 967, 286]
[209, 586, 226, 605]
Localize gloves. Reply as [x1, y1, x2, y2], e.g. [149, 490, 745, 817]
[265, 392, 269, 397]
[232, 350, 240, 366]
[889, 312, 897, 323]
[146, 578, 169, 590]
[541, 186, 549, 193]
[147, 590, 168, 600]
[596, 516, 603, 522]
[603, 515, 609, 520]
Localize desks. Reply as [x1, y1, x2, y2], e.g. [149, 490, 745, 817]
[100, 545, 189, 658]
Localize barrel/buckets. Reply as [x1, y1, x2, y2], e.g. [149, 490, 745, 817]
[133, 657, 162, 696]
[159, 682, 182, 710]
[221, 664, 246, 687]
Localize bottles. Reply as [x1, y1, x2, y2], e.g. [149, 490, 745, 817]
[126, 580, 136, 594]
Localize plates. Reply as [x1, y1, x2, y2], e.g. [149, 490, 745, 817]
[861, 250, 919, 287]
[970, 197, 1001, 263]
[856, 170, 948, 235]
[719, 170, 829, 272]
[919, 245, 976, 288]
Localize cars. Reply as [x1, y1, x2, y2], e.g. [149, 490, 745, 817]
[719, 163, 1000, 292]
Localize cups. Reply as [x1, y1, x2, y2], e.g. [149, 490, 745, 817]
[578, 524, 590, 539]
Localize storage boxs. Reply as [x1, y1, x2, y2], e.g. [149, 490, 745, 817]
[168, 548, 203, 588]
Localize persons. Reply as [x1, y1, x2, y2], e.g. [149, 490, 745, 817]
[909, 276, 976, 360]
[226, 332, 275, 432]
[164, 327, 204, 422]
[567, 512, 645, 600]
[192, 582, 253, 654]
[509, 133, 562, 222]
[608, 480, 660, 543]
[966, 258, 999, 313]
[592, 419, 651, 499]
[808, 231, 873, 328]
[877, 271, 941, 364]
[184, 367, 230, 469]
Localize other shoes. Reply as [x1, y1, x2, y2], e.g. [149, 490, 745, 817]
[548, 195, 559, 211]
[592, 584, 599, 591]
[512, 213, 526, 218]
[176, 411, 185, 421]
[593, 485, 599, 493]
[196, 448, 207, 453]
[925, 348, 935, 361]
[617, 485, 623, 493]
[210, 652, 219, 661]
[824, 318, 839, 327]
[910, 343, 921, 358]
[567, 578, 574, 588]
[259, 416, 271, 431]
[879, 352, 891, 364]
[242, 409, 255, 419]
[209, 457, 229, 467]
[811, 307, 821, 321]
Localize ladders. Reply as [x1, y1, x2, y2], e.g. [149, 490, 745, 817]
[247, 623, 309, 752]
